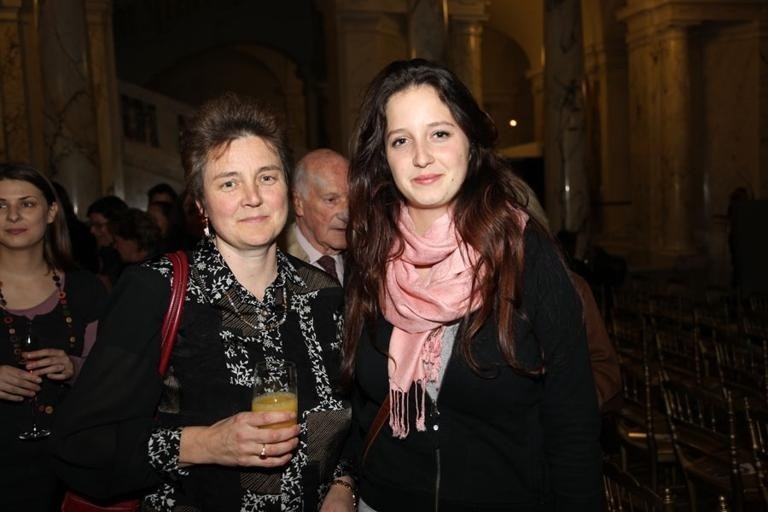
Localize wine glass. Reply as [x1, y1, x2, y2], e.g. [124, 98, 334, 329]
[13, 333, 51, 446]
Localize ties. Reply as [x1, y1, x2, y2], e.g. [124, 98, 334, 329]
[318, 255, 338, 281]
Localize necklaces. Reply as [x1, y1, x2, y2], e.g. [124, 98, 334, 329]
[1, 261, 76, 418]
[220, 288, 287, 330]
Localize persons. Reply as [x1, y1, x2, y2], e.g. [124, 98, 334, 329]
[53, 183, 186, 295]
[64, 91, 352, 510]
[282, 149, 352, 290]
[1, 170, 108, 511]
[315, 58, 625, 511]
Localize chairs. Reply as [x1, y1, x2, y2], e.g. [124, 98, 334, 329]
[592, 286, 768, 512]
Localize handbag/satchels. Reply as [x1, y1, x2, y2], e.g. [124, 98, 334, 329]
[64, 255, 187, 511]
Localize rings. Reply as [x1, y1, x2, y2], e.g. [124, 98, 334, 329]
[259, 443, 266, 459]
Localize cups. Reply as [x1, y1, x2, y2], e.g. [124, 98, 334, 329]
[252, 362, 299, 433]
